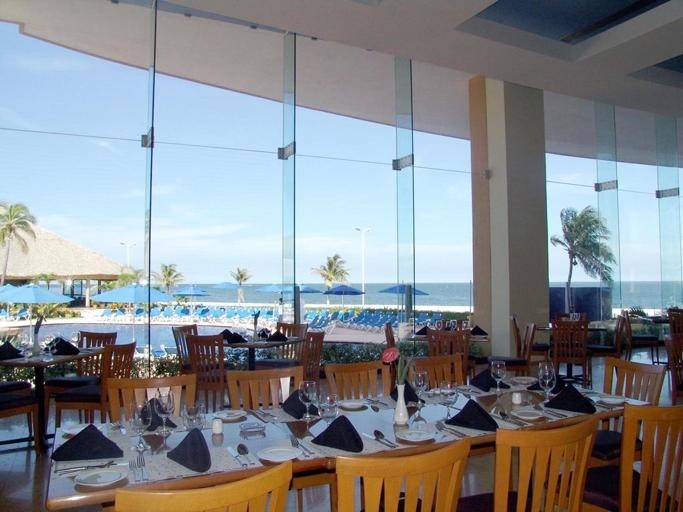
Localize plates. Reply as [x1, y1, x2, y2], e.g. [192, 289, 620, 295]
[599, 398, 624, 405]
[513, 411, 543, 417]
[395, 430, 432, 441]
[240, 421, 265, 432]
[256, 445, 301, 463]
[340, 399, 363, 409]
[510, 377, 536, 385]
[74, 467, 127, 486]
[61, 425, 104, 435]
[213, 410, 246, 420]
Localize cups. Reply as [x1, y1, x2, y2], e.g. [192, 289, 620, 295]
[511, 392, 520, 404]
[183, 403, 204, 433]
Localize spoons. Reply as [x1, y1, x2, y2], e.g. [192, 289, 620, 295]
[374, 429, 398, 446]
[498, 410, 528, 425]
[237, 443, 254, 464]
[596, 400, 616, 409]
[251, 408, 276, 422]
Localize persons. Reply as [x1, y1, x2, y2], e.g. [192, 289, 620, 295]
[277, 295, 282, 332]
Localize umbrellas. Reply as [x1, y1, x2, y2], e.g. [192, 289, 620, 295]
[256, 283, 283, 306]
[89, 283, 170, 343]
[322, 284, 365, 309]
[171, 281, 210, 311]
[283, 285, 322, 310]
[0, 282, 74, 342]
[210, 281, 240, 299]
[0, 282, 17, 322]
[377, 283, 430, 320]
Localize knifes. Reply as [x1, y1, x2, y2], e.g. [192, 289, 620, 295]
[362, 432, 395, 448]
[228, 446, 247, 465]
[367, 398, 387, 406]
[54, 460, 115, 473]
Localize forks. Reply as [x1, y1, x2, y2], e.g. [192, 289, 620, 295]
[533, 403, 567, 417]
[129, 455, 148, 482]
[435, 421, 468, 437]
[289, 434, 314, 457]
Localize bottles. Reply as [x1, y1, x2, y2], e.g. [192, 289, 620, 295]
[211, 418, 223, 433]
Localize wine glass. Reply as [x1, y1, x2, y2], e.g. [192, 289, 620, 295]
[154, 391, 174, 435]
[15, 329, 82, 361]
[537, 362, 556, 404]
[413, 371, 429, 407]
[431, 318, 469, 333]
[318, 394, 337, 428]
[229, 317, 283, 343]
[298, 381, 316, 418]
[130, 400, 151, 449]
[490, 360, 506, 392]
[439, 381, 457, 419]
